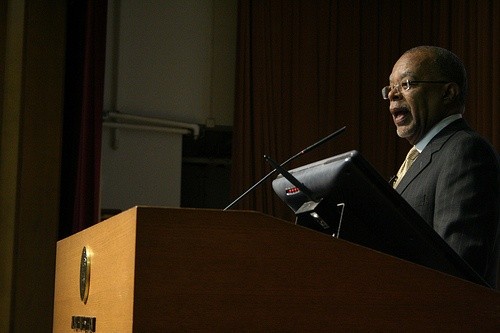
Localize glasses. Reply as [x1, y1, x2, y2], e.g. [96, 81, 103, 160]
[382, 78, 459, 99]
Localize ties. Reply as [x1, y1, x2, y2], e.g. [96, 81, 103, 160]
[393, 145, 420, 190]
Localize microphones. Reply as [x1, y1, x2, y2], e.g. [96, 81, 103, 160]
[224, 127, 348, 210]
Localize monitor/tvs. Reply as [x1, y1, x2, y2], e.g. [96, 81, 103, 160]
[273, 150, 491, 289]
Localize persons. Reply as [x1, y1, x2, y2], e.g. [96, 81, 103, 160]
[381, 45, 500, 291]
